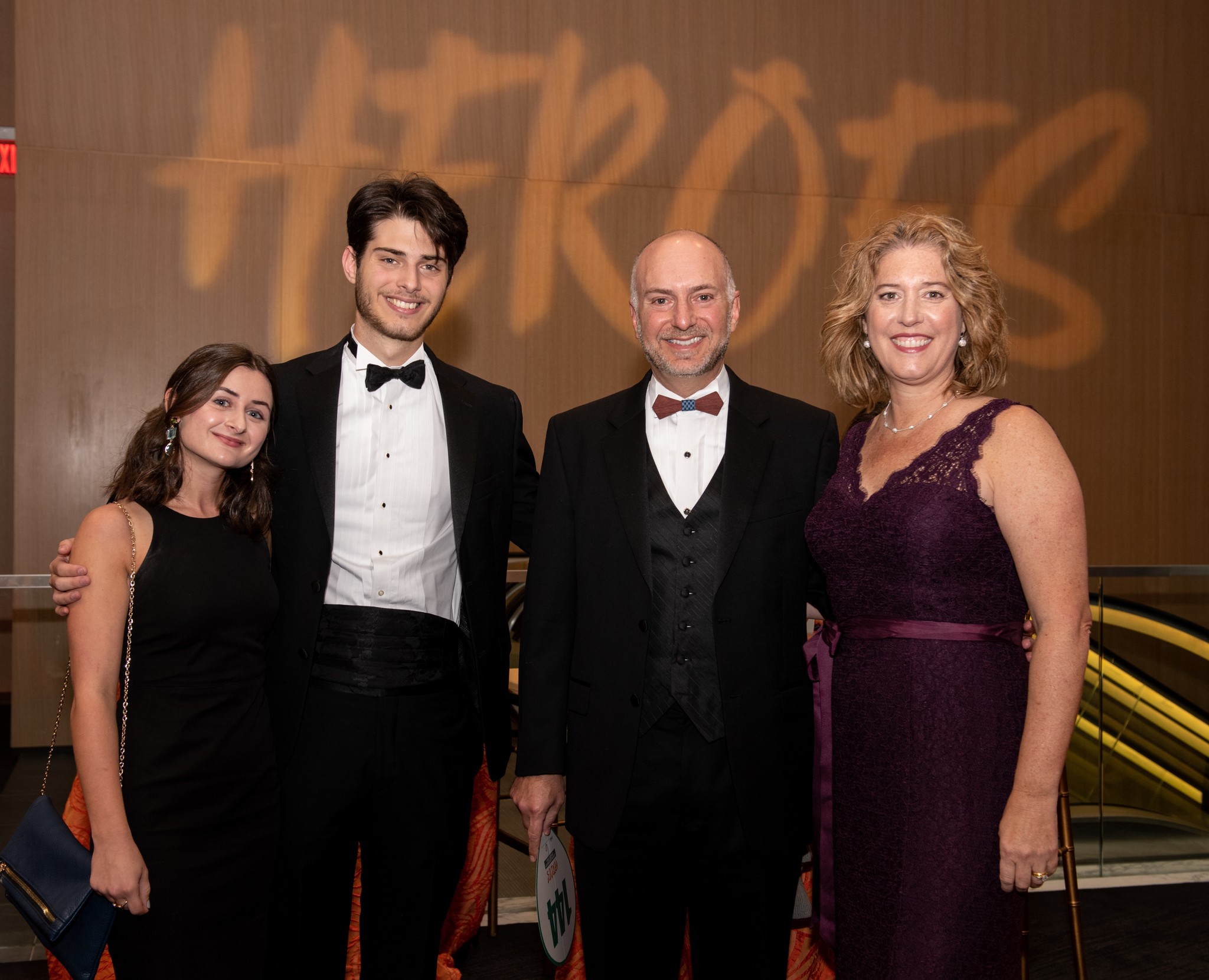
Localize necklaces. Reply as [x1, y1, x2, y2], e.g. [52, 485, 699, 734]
[884, 397, 960, 434]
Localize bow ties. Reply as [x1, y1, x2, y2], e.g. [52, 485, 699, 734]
[652, 391, 724, 420]
[347, 329, 425, 392]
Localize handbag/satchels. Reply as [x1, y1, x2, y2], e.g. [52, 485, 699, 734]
[0, 795, 116, 980]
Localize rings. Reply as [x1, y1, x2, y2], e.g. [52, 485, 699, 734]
[113, 901, 128, 908]
[1031, 871, 1048, 882]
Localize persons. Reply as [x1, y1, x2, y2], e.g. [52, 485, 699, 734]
[507, 229, 1036, 980]
[805, 211, 1093, 980]
[49, 175, 541, 980]
